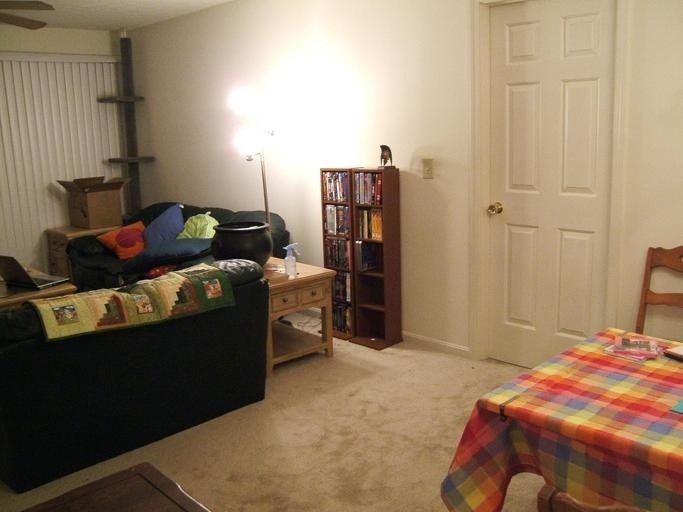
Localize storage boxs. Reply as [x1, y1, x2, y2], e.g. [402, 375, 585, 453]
[57, 176, 132, 230]
[45, 225, 123, 282]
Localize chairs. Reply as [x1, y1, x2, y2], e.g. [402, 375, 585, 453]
[538, 485, 648, 512]
[636, 245, 683, 334]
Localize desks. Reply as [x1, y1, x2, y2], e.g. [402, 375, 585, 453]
[441, 325, 683, 512]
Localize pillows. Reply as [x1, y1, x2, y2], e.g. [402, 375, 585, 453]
[96, 221, 146, 261]
[143, 203, 185, 243]
[136, 239, 214, 264]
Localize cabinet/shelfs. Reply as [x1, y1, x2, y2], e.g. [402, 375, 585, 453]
[321, 166, 404, 350]
[98, 37, 155, 213]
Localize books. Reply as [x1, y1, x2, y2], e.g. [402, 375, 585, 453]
[613, 335, 658, 358]
[603, 344, 648, 362]
[320, 170, 384, 336]
[662, 344, 683, 362]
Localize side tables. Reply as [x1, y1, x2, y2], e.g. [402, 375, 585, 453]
[264, 255, 337, 378]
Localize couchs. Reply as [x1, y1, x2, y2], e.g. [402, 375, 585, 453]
[66, 202, 291, 290]
[0, 258, 269, 494]
